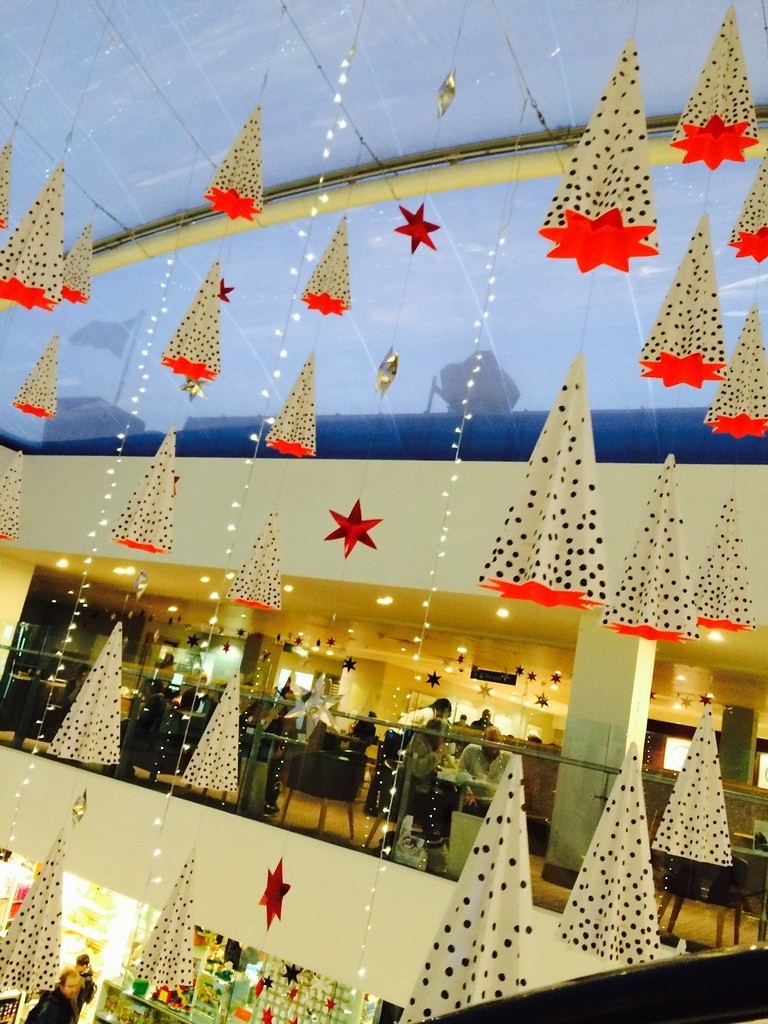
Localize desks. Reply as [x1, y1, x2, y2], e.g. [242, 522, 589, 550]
[120, 687, 146, 702]
[434, 763, 499, 813]
[178, 710, 208, 720]
[728, 843, 768, 945]
[245, 726, 311, 818]
[8, 672, 68, 689]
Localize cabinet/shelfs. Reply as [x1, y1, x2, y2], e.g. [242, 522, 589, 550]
[92, 975, 215, 1024]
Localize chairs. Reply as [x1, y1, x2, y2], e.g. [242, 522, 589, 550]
[0, 660, 551, 886]
[658, 850, 751, 949]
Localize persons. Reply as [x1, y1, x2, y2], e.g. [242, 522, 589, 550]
[403, 718, 450, 848]
[24, 953, 97, 1024]
[440, 726, 514, 837]
[121, 678, 164, 781]
[362, 698, 452, 822]
[59, 664, 93, 731]
[453, 715, 469, 728]
[471, 709, 493, 731]
[349, 710, 379, 782]
